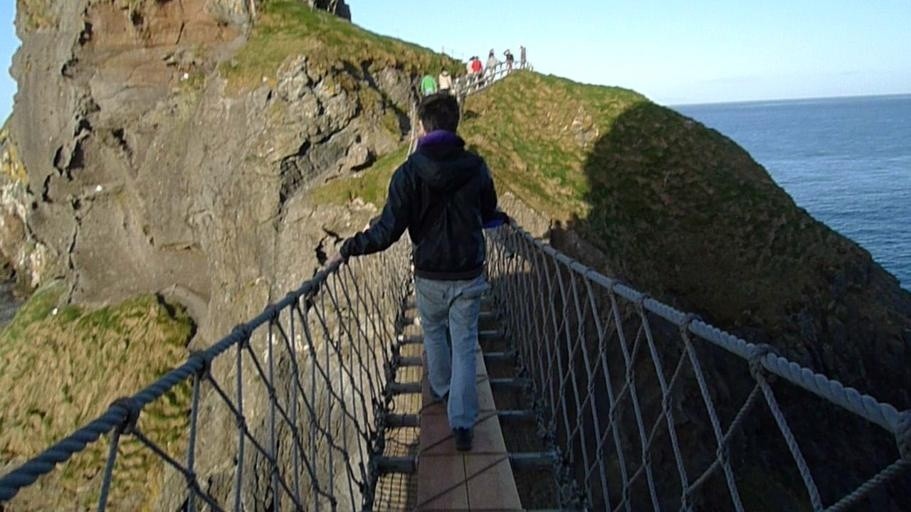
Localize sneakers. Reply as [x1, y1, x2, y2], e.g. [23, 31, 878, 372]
[455, 425, 474, 451]
[429, 385, 450, 406]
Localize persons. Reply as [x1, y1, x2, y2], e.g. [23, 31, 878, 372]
[328, 90, 523, 451]
[466, 45, 526, 88]
[439, 65, 453, 94]
[421, 70, 438, 97]
